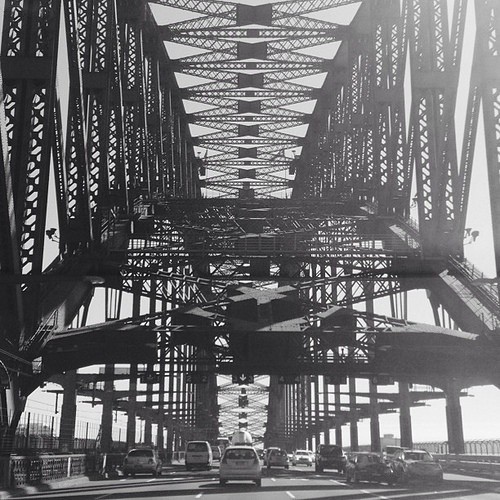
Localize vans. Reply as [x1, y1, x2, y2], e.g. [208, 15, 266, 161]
[210, 444, 221, 459]
[185, 439, 213, 470]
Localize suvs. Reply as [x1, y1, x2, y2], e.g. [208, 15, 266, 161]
[388, 450, 444, 486]
[219, 445, 263, 486]
[315, 445, 347, 472]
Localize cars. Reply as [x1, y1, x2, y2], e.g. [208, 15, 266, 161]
[266, 449, 289, 469]
[122, 447, 163, 478]
[292, 450, 312, 467]
[345, 452, 386, 482]
[264, 446, 281, 465]
